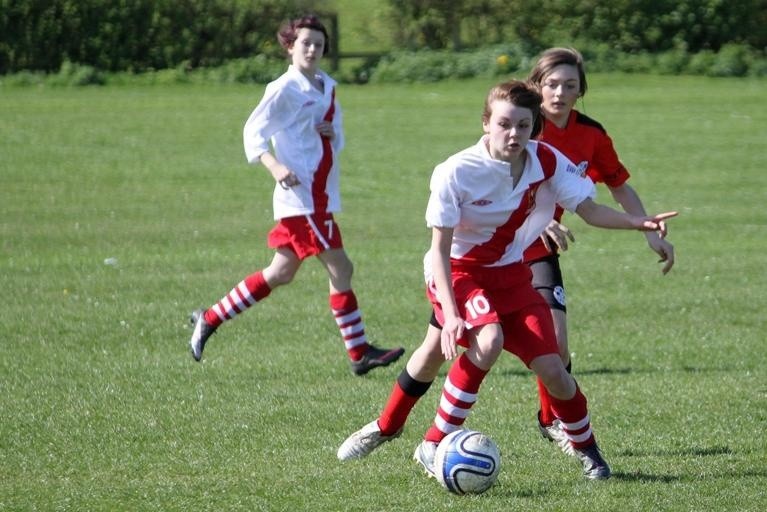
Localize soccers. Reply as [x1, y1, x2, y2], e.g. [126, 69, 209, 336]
[434, 429, 500, 495]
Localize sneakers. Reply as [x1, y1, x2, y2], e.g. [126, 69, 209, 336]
[350, 344, 405, 377]
[538, 410, 610, 480]
[337, 418, 403, 465]
[189, 309, 216, 362]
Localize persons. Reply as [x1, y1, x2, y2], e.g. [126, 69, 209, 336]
[190, 14, 404, 376]
[337, 47, 676, 464]
[414, 82, 678, 478]
